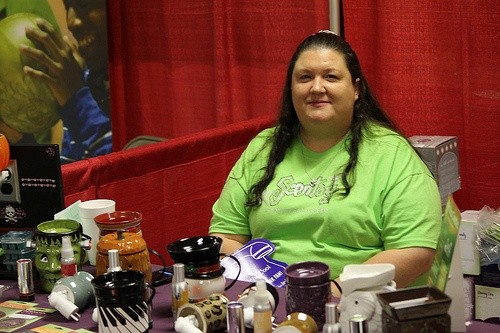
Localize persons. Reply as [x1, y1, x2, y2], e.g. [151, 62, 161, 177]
[0, 0, 113, 166]
[208, 28, 443, 299]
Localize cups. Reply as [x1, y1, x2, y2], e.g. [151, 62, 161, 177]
[34, 199, 241, 333]
[284, 259, 332, 333]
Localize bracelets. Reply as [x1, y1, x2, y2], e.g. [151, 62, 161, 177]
[329, 279, 342, 294]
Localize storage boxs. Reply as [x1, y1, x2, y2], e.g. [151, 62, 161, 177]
[407, 135, 461, 198]
